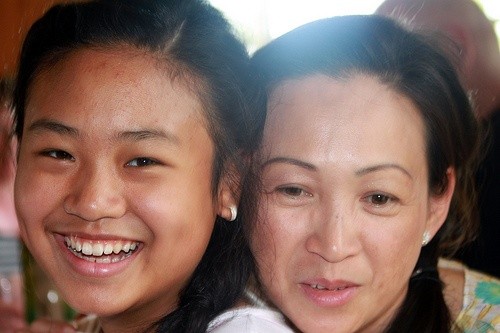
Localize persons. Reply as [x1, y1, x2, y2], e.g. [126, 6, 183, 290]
[376, 0, 500, 278]
[240, 14, 500, 333]
[0, 0, 298, 333]
